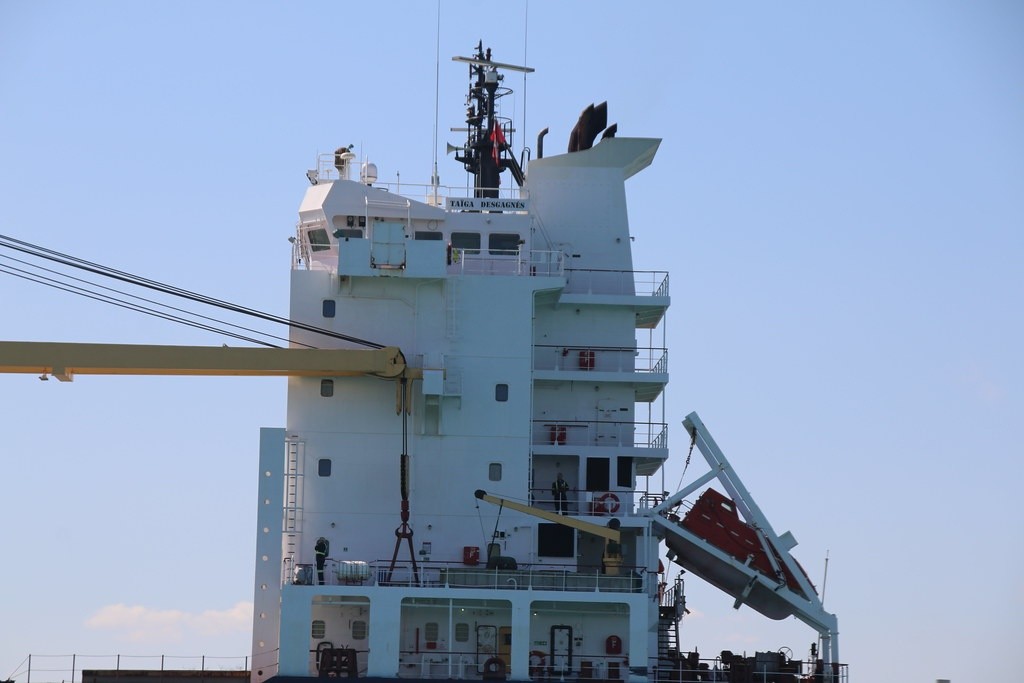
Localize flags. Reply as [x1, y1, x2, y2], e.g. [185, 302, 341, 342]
[492, 122, 507, 167]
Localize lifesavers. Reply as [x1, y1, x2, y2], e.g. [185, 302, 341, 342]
[530, 651, 545, 667]
[599, 493, 621, 514]
[484, 657, 506, 679]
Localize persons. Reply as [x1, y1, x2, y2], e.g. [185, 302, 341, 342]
[551, 472, 569, 517]
[314, 536, 328, 586]
[334, 143, 354, 180]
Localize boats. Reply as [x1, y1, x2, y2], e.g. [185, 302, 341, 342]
[83, 0, 849, 683]
